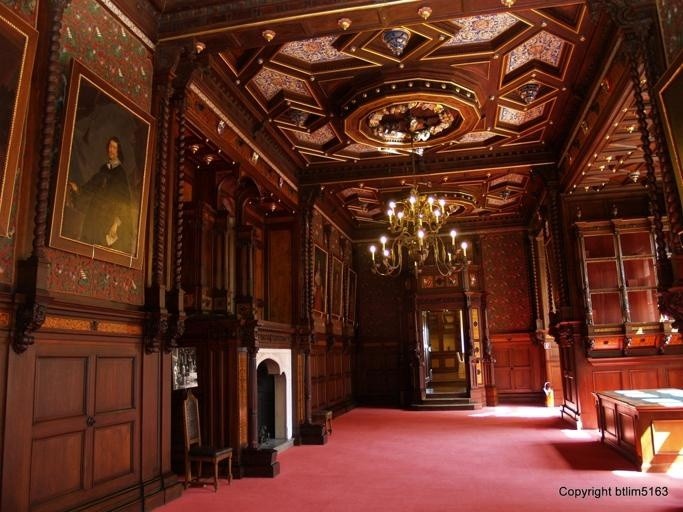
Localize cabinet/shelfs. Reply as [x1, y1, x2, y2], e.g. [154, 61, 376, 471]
[572, 215, 680, 336]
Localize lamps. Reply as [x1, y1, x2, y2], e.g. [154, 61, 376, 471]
[363, 109, 475, 278]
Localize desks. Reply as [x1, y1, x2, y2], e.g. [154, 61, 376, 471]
[589, 388, 683, 473]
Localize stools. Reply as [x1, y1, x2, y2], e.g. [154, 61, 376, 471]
[312, 409, 333, 437]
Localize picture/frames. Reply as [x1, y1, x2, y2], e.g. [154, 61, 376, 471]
[46, 55, 158, 271]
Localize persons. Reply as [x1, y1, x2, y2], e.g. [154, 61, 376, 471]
[66, 135, 134, 255]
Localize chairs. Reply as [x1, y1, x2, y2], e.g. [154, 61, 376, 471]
[182, 388, 235, 492]
